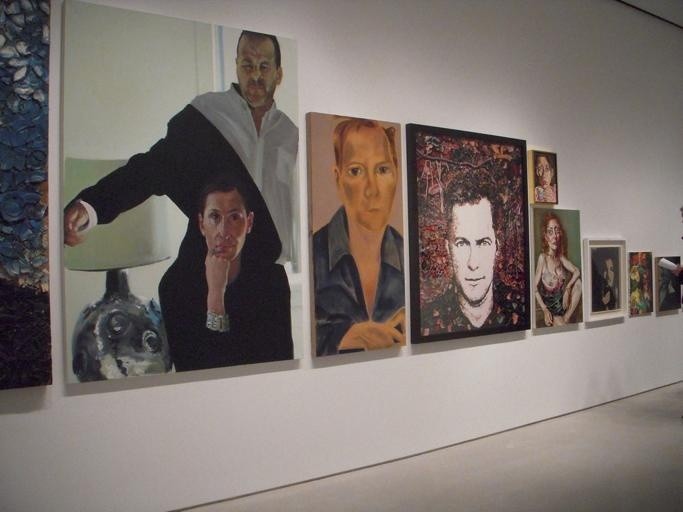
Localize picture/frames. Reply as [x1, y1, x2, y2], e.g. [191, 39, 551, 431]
[402, 122, 533, 344]
[581, 232, 629, 328]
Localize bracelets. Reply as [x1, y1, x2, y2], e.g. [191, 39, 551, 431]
[203, 311, 231, 334]
[564, 286, 571, 295]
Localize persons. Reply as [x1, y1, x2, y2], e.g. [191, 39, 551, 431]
[160, 170, 295, 371]
[534, 212, 582, 327]
[314, 117, 405, 359]
[533, 154, 557, 204]
[593, 252, 619, 313]
[666, 205, 683, 288]
[418, 167, 525, 339]
[62, 31, 301, 272]
